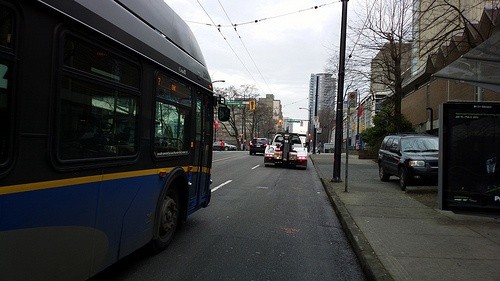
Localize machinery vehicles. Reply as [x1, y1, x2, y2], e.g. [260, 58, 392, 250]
[263, 128, 309, 170]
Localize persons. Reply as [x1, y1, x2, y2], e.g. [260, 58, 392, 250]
[317, 140, 322, 154]
[220, 140, 224, 151]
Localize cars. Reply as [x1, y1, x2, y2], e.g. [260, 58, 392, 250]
[212, 141, 236, 151]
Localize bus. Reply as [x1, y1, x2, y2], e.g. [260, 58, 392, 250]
[0, 0, 231, 281]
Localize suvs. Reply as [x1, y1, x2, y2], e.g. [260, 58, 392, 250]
[377, 133, 440, 191]
[249, 138, 268, 155]
